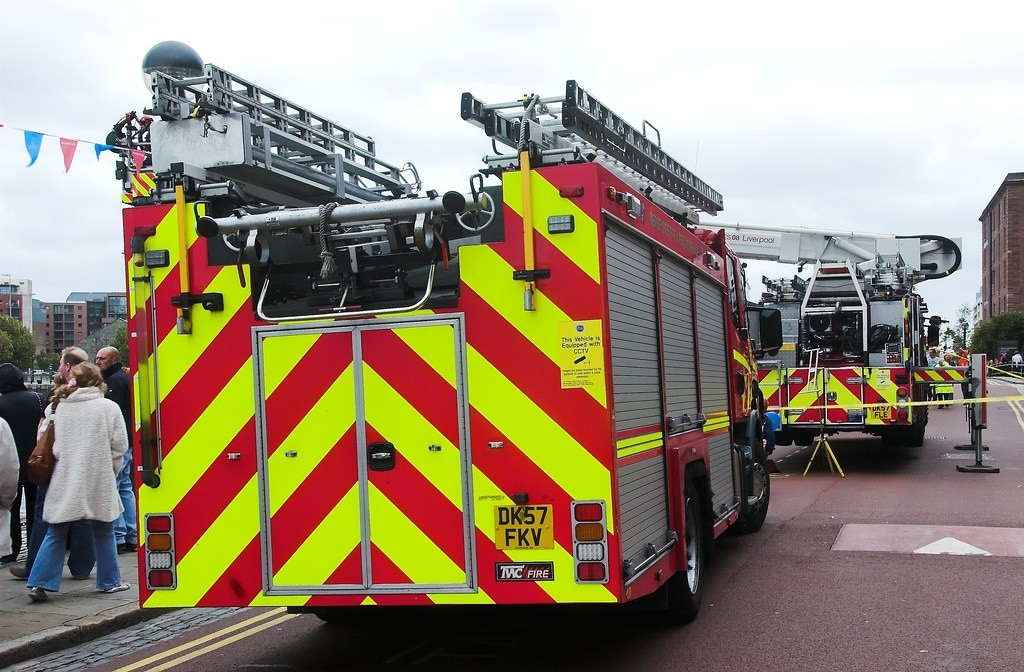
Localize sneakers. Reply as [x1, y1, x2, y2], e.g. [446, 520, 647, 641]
[116, 541, 137, 554]
[10, 564, 29, 578]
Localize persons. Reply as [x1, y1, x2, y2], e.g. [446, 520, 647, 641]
[36, 372, 66, 445]
[760, 397, 775, 457]
[924, 346, 1024, 409]
[26, 362, 131, 604]
[1, 363, 50, 565]
[0, 417, 20, 560]
[93, 346, 140, 555]
[10, 345, 102, 578]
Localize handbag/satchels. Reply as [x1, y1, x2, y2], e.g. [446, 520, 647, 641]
[23, 394, 61, 485]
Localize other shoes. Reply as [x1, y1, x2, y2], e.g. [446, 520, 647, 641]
[105, 580, 131, 595]
[24, 586, 51, 602]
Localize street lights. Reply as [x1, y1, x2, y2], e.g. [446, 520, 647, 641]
[961, 323, 970, 354]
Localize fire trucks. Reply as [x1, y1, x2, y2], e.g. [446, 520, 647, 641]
[104, 38, 779, 624]
[679, 222, 1002, 483]
